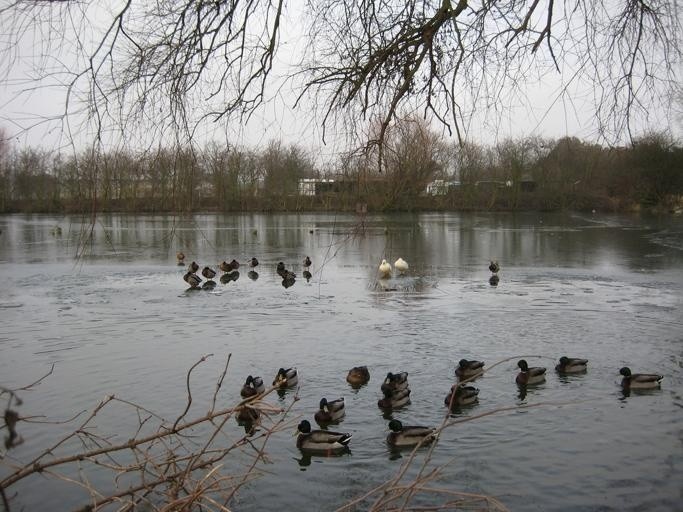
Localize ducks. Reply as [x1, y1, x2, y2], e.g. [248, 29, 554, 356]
[234, 366, 300, 437]
[615, 366, 665, 391]
[346, 364, 370, 385]
[292, 395, 354, 454]
[444, 359, 485, 410]
[376, 369, 439, 449]
[512, 353, 588, 387]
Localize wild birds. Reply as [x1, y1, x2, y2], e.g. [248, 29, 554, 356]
[177, 252, 185, 262]
[277, 261, 296, 279]
[379, 257, 409, 278]
[182, 256, 260, 291]
[489, 260, 500, 275]
[302, 256, 312, 269]
